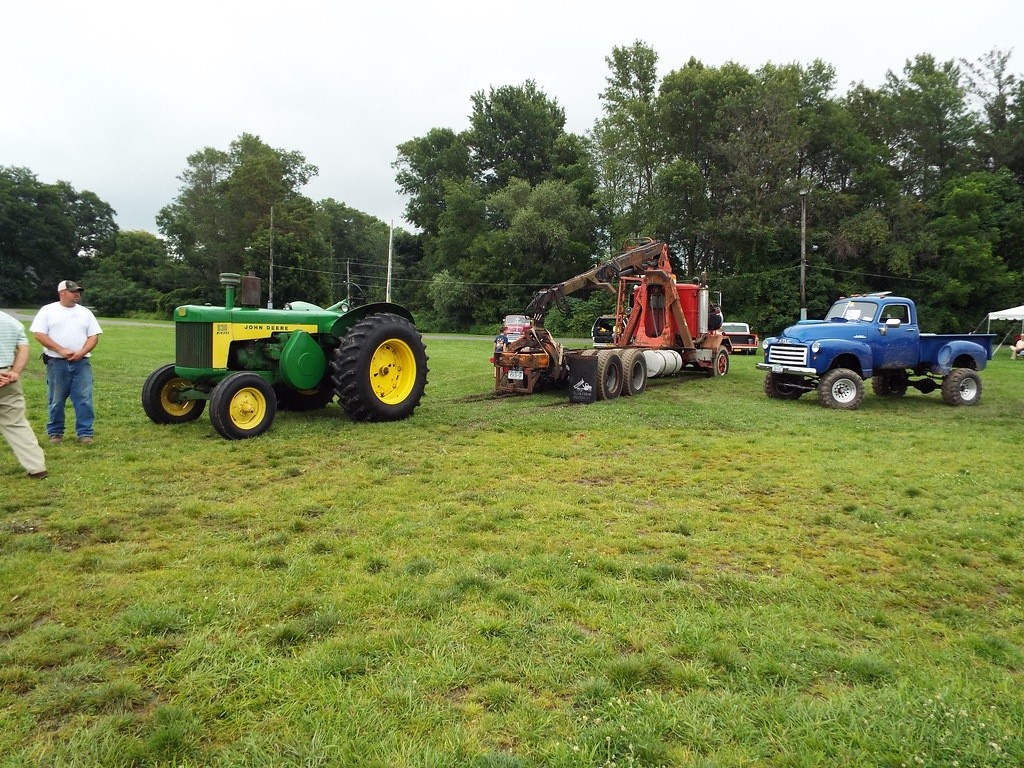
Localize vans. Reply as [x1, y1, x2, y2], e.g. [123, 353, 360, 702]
[591, 315, 630, 348]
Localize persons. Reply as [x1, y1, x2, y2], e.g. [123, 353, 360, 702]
[494, 327, 508, 378]
[29, 280, 103, 443]
[1009, 334, 1024, 360]
[0, 310, 50, 480]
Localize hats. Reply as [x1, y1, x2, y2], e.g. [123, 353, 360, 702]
[57, 280, 83, 292]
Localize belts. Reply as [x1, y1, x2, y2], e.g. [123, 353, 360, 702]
[0, 366, 13, 369]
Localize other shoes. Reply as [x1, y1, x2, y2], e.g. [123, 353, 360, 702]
[29, 472, 47, 479]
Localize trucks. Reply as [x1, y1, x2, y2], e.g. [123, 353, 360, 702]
[755, 291, 999, 410]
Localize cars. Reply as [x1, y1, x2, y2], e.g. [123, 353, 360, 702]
[719, 321, 760, 356]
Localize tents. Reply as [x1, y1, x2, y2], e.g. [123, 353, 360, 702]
[987, 306, 1024, 336]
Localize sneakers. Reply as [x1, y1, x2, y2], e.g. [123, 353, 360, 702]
[80, 435, 94, 444]
[49, 434, 63, 443]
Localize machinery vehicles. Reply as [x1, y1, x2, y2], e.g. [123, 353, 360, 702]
[490, 235, 734, 403]
[142, 269, 431, 441]
